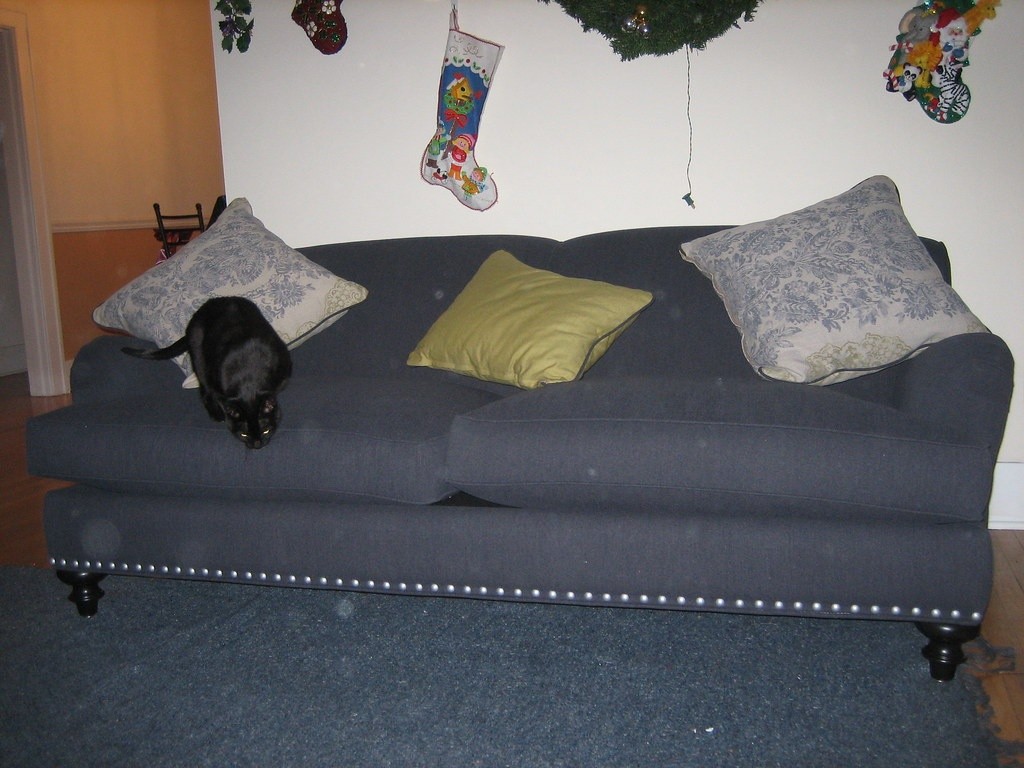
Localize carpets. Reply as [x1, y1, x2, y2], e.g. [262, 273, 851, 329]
[0, 566, 1024, 768]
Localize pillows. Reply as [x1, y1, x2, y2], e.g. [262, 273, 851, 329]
[90, 195, 370, 389]
[678, 175, 992, 385]
[405, 250, 654, 390]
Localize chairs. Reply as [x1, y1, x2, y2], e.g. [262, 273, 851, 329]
[153, 203, 204, 258]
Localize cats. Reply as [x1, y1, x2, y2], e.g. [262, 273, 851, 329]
[120, 296, 292, 449]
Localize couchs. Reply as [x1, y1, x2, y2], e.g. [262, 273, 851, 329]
[26, 226, 1016, 683]
